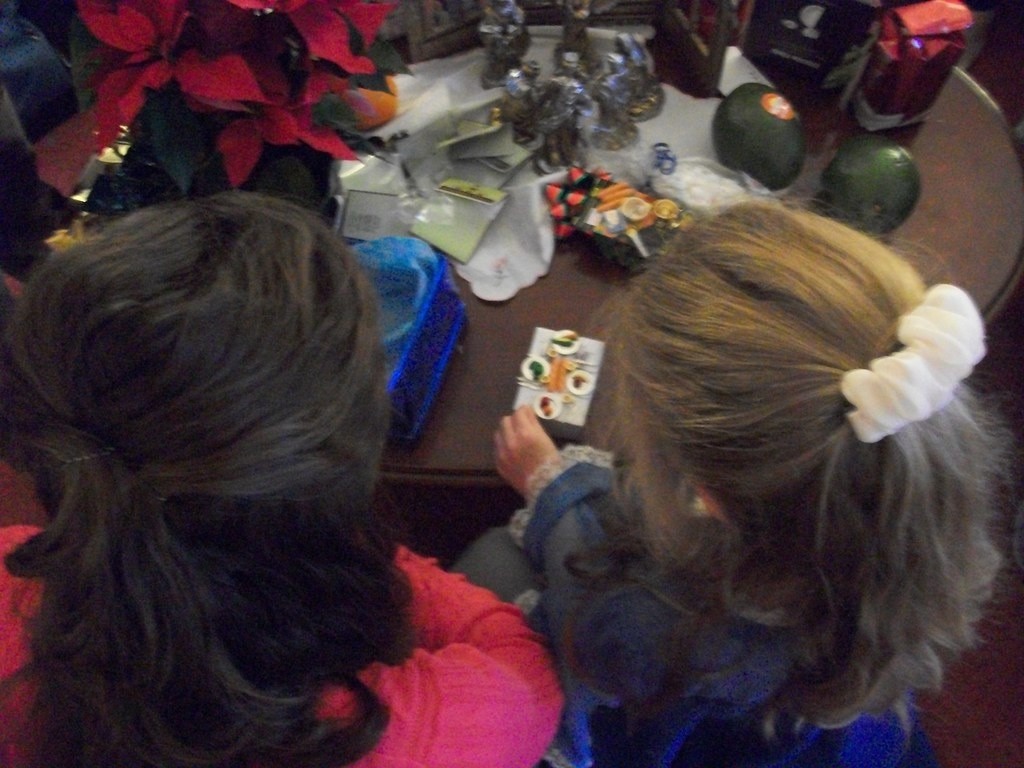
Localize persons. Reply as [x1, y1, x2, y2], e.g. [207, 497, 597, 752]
[491, 203, 1012, 767]
[4, 194, 562, 768]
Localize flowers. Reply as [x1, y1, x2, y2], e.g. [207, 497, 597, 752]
[69, 0, 418, 195]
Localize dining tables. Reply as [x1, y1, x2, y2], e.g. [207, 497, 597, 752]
[330, 0, 1023, 496]
